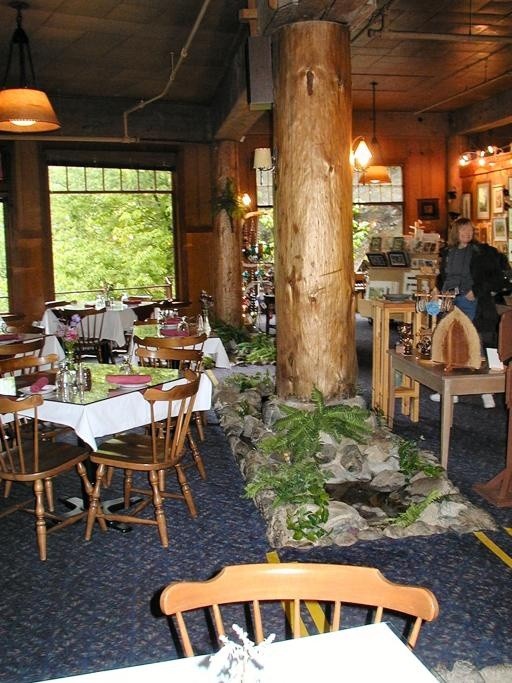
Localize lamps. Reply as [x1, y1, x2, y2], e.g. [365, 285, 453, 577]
[252, 145, 278, 186]
[359, 81, 393, 185]
[0, 2, 63, 135]
[242, 193, 252, 212]
[350, 135, 371, 172]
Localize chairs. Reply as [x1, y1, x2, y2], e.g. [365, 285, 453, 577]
[157, 560, 440, 654]
[50, 307, 107, 364]
[85, 368, 201, 548]
[134, 347, 204, 371]
[1, 338, 43, 382]
[0, 353, 60, 385]
[0, 393, 107, 561]
[132, 333, 207, 370]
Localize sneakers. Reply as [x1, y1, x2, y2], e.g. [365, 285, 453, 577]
[481, 395, 495, 408]
[429, 393, 458, 404]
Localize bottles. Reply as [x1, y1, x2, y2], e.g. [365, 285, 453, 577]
[75, 362, 85, 388]
[198, 316, 203, 330]
[55, 361, 72, 391]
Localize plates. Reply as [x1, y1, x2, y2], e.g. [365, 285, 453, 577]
[18, 385, 55, 394]
[117, 383, 146, 387]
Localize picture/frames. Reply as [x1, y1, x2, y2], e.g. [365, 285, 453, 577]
[492, 217, 507, 243]
[369, 236, 382, 251]
[476, 180, 491, 220]
[492, 184, 504, 214]
[416, 198, 440, 220]
[366, 252, 388, 267]
[392, 237, 404, 250]
[387, 251, 409, 267]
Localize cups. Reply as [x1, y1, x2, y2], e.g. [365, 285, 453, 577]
[119, 354, 134, 375]
[56, 361, 73, 396]
[177, 317, 189, 337]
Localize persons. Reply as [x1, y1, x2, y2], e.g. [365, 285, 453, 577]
[429, 217, 496, 409]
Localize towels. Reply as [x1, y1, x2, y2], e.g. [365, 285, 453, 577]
[106, 375, 151, 384]
[85, 305, 95, 308]
[0, 334, 18, 341]
[30, 377, 49, 392]
[160, 329, 188, 336]
[123, 300, 141, 304]
[167, 320, 179, 324]
